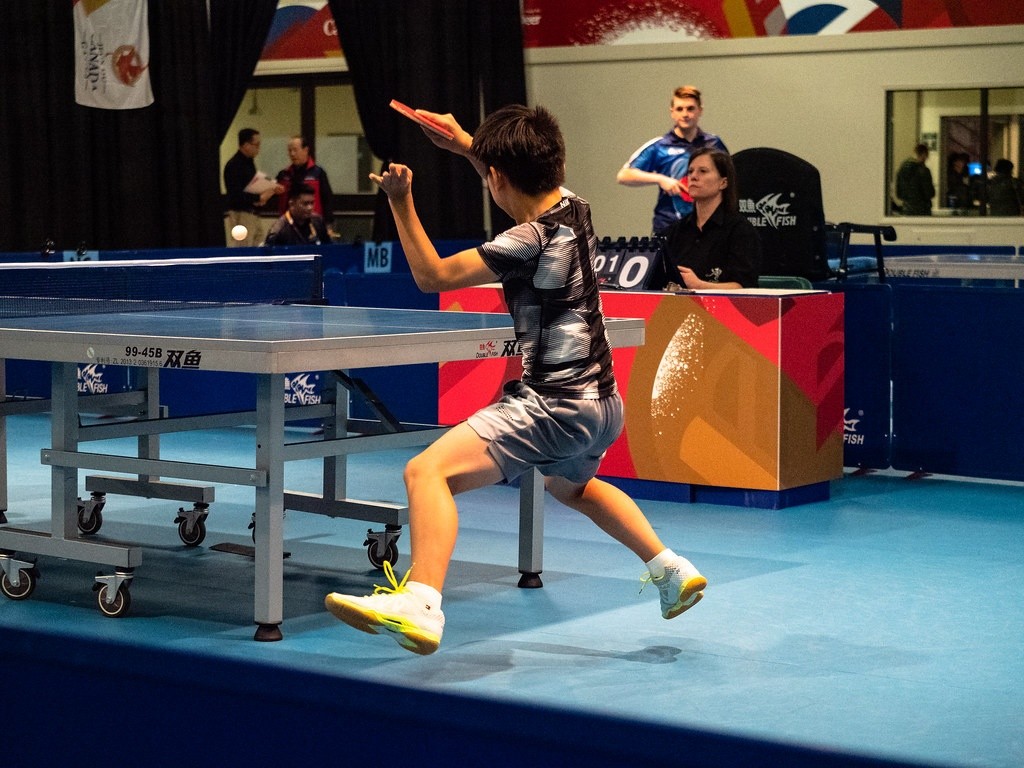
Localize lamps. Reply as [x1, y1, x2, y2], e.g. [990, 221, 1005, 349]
[249, 90, 264, 115]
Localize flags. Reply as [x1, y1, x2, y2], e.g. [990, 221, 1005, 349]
[73, 0, 154, 110]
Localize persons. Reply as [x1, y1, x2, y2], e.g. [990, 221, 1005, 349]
[614, 86, 732, 240]
[323, 102, 709, 656]
[895, 144, 936, 216]
[985, 158, 1024, 216]
[276, 135, 337, 239]
[641, 146, 759, 295]
[263, 182, 334, 248]
[224, 128, 285, 216]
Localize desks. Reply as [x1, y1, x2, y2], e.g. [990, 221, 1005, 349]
[0, 255, 545, 641]
[438, 283, 845, 510]
[884, 254, 1024, 288]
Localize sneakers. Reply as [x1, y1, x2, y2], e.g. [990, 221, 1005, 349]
[638, 556, 706, 620]
[324, 561, 445, 655]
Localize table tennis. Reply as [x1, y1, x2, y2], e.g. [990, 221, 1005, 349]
[232, 224, 248, 240]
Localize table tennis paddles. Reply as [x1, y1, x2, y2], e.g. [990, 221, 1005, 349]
[389, 99, 455, 145]
